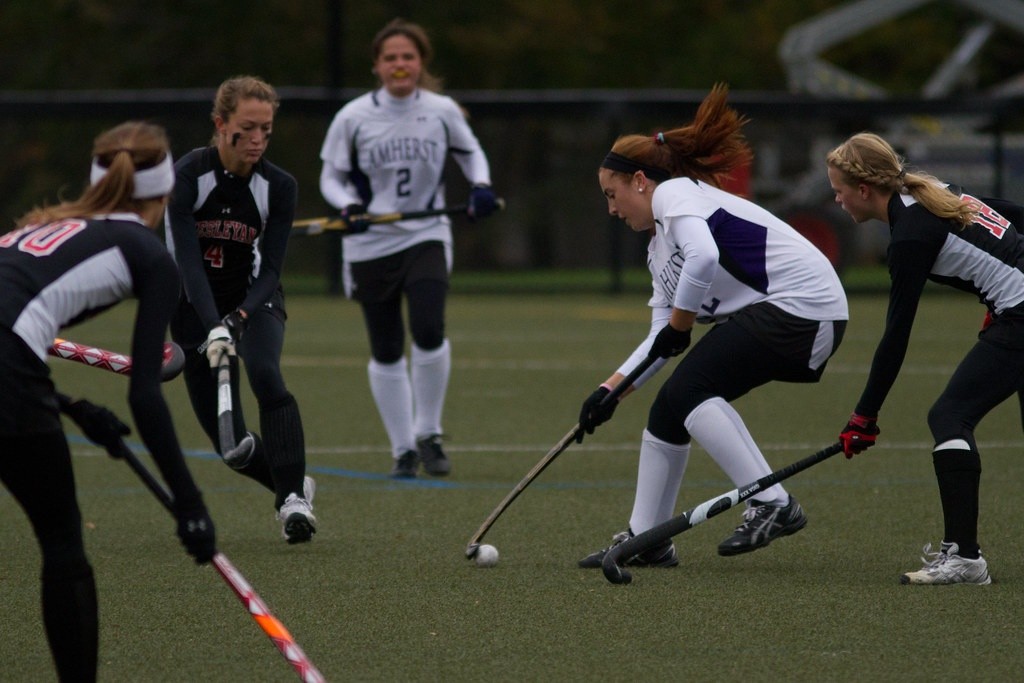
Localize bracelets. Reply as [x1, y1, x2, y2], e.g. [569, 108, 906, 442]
[599, 383, 621, 400]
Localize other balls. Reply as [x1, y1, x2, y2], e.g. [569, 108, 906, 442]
[475, 543, 500, 568]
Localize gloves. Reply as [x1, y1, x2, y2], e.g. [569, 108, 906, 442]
[576, 384, 619, 443]
[467, 189, 505, 216]
[839, 411, 880, 459]
[221, 310, 246, 337]
[207, 338, 238, 369]
[339, 205, 370, 235]
[70, 400, 129, 459]
[177, 500, 216, 565]
[647, 323, 692, 360]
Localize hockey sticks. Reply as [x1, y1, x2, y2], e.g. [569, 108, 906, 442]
[601, 443, 844, 584]
[288, 197, 506, 240]
[125, 446, 324, 683]
[216, 353, 265, 472]
[463, 354, 654, 560]
[48, 334, 186, 383]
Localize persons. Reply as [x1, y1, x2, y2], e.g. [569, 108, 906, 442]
[0, 121, 217, 683]
[575, 81, 849, 568]
[827, 134, 1024, 586]
[320, 18, 497, 478]
[164, 76, 316, 545]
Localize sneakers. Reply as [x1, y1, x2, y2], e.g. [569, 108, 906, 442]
[718, 494, 808, 555]
[391, 450, 418, 479]
[577, 529, 678, 569]
[416, 434, 451, 477]
[302, 476, 316, 500]
[276, 493, 318, 544]
[901, 539, 992, 586]
